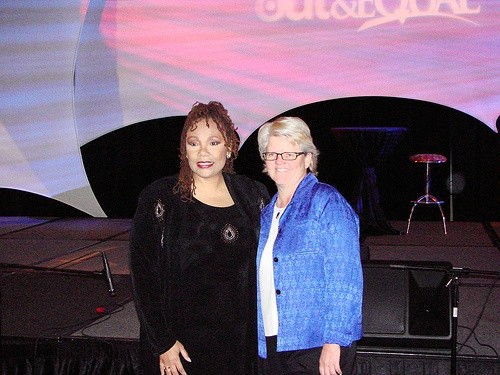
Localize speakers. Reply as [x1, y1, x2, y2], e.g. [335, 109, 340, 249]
[356, 259, 458, 349]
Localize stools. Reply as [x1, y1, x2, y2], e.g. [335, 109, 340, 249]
[407, 153, 449, 236]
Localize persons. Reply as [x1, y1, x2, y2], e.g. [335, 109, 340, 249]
[255, 117, 363, 375]
[128, 102, 273, 375]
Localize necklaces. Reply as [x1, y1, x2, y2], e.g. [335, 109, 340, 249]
[276, 200, 289, 219]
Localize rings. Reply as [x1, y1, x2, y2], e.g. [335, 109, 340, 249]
[163, 366, 170, 370]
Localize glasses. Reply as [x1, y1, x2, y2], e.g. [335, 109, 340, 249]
[262, 152, 304, 161]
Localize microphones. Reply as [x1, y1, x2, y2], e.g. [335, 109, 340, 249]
[102, 251, 117, 296]
